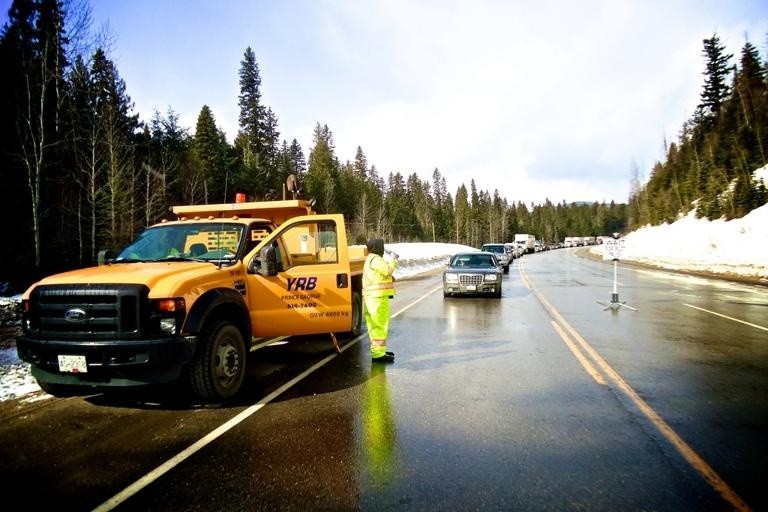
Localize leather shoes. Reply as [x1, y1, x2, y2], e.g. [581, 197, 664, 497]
[374, 352, 394, 362]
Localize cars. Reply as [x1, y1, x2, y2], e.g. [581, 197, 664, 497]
[482, 243, 510, 274]
[442, 250, 504, 298]
[507, 233, 607, 259]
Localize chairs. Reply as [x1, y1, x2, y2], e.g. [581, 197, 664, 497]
[190, 243, 207, 257]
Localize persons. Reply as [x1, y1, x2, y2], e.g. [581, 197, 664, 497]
[360, 239, 400, 362]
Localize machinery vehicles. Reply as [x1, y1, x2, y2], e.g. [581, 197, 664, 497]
[13, 192, 371, 400]
